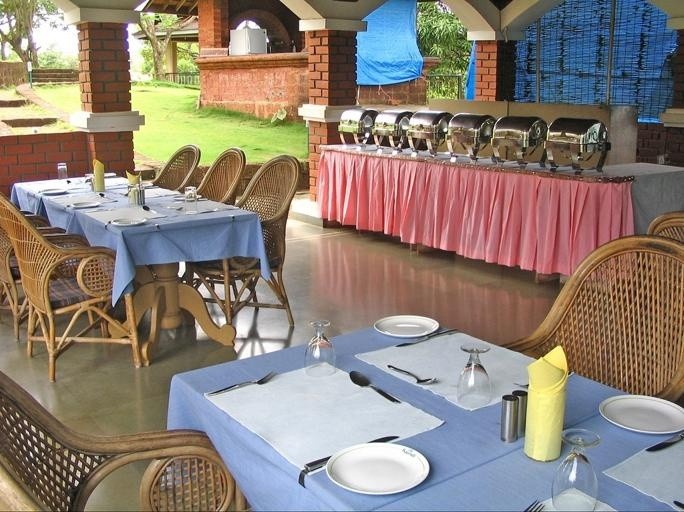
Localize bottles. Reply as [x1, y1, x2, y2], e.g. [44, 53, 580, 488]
[500, 395, 518, 444]
[511, 389, 527, 437]
[127, 184, 144, 207]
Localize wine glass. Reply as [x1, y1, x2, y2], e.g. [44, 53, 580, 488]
[550, 428, 602, 510]
[303, 319, 336, 377]
[455, 341, 492, 407]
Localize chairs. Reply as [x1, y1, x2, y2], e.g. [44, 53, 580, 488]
[503, 233, 684, 408]
[184, 154, 302, 329]
[645, 208, 683, 242]
[0, 210, 51, 311]
[153, 145, 201, 192]
[0, 194, 143, 383]
[1, 227, 109, 344]
[0, 369, 250, 511]
[195, 146, 247, 205]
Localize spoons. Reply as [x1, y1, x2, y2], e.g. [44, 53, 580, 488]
[141, 205, 157, 214]
[347, 369, 399, 404]
[98, 192, 116, 202]
[387, 364, 435, 384]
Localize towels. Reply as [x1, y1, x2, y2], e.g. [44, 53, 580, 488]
[526, 342, 571, 466]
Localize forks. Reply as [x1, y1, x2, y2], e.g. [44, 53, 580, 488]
[512, 369, 574, 389]
[205, 369, 275, 396]
[524, 498, 546, 511]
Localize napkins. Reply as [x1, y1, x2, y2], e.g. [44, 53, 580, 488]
[93, 159, 104, 192]
[126, 170, 143, 184]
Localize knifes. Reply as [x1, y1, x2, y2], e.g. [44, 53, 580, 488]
[645, 432, 684, 451]
[304, 434, 400, 473]
[395, 328, 459, 346]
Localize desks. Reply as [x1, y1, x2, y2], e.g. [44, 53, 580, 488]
[317, 144, 684, 289]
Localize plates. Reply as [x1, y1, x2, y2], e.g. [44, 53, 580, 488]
[110, 216, 147, 227]
[174, 195, 200, 201]
[598, 394, 683, 434]
[40, 188, 66, 194]
[323, 442, 430, 494]
[72, 201, 100, 208]
[372, 315, 439, 337]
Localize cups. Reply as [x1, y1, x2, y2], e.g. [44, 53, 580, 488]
[83, 173, 95, 195]
[184, 186, 198, 215]
[56, 163, 67, 182]
